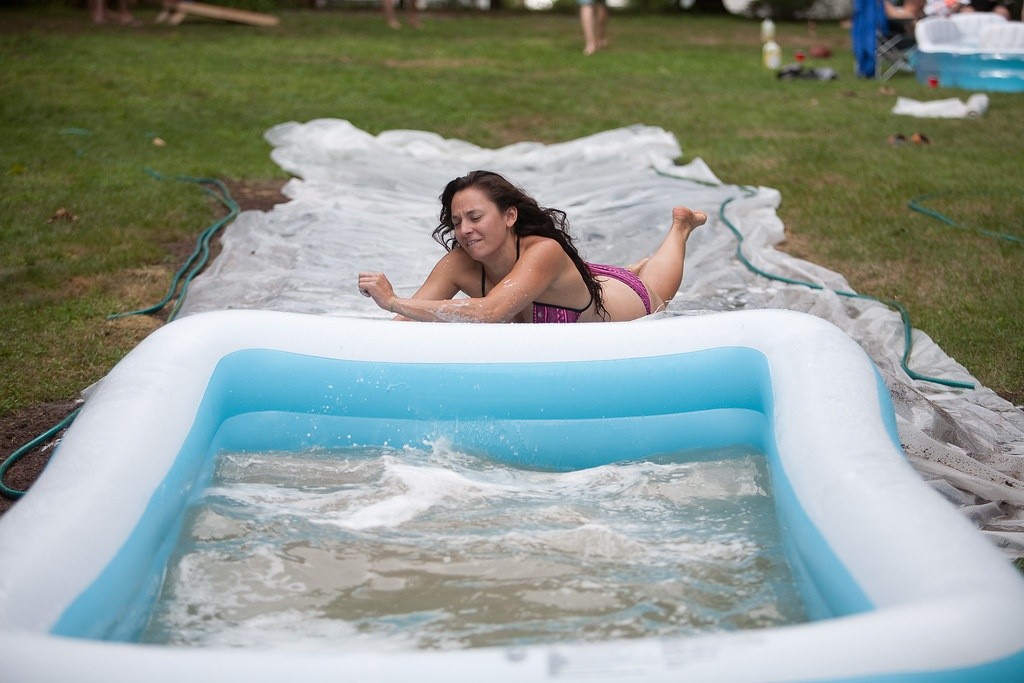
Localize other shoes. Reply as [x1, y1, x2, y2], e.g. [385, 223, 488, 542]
[888, 134, 934, 144]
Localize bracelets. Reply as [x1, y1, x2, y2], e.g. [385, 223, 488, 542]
[390, 296, 399, 313]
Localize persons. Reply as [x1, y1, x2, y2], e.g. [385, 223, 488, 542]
[356, 170, 707, 324]
[382, 0, 426, 30]
[880, 0, 1024, 73]
[92, 0, 134, 24]
[576, 0, 608, 57]
